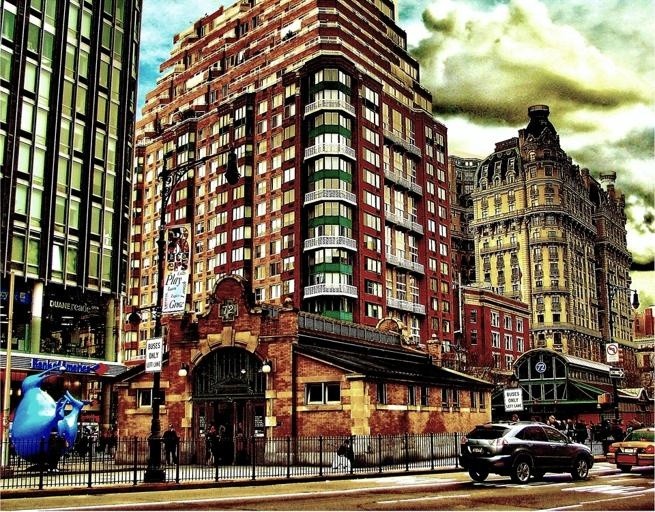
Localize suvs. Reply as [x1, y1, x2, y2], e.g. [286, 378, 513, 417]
[458, 419, 595, 485]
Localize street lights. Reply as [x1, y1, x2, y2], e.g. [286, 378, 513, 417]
[142, 145, 241, 483]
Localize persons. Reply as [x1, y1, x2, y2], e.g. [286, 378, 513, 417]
[162, 423, 179, 465]
[205, 424, 219, 467]
[235, 422, 244, 440]
[333, 439, 355, 471]
[546, 415, 646, 456]
[512, 413, 520, 421]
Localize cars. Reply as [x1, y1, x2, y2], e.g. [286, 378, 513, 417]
[605, 427, 655, 472]
